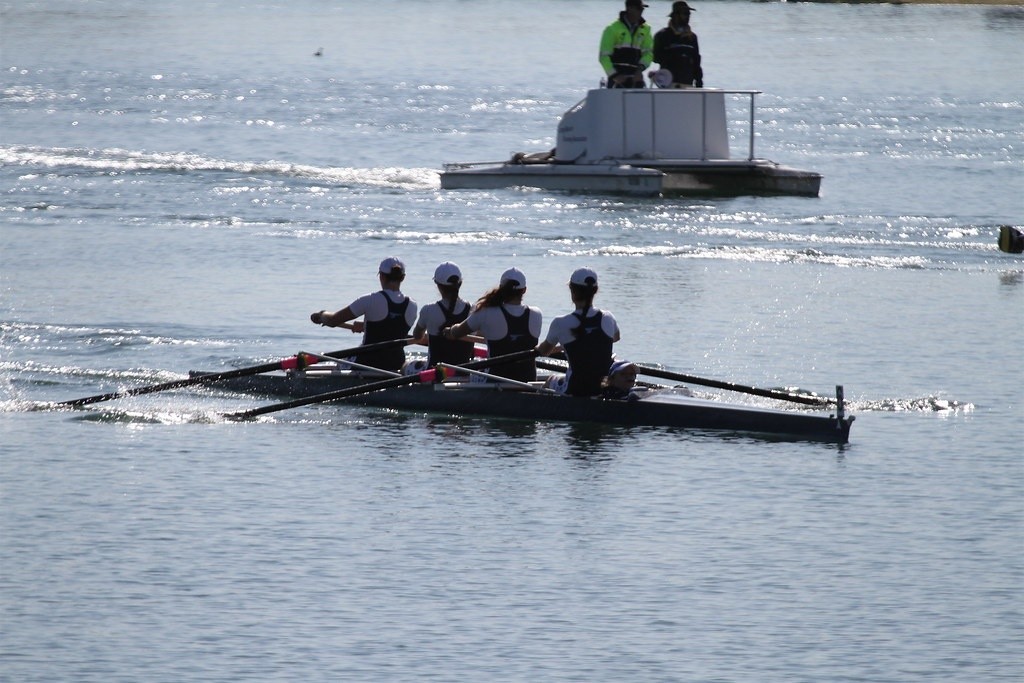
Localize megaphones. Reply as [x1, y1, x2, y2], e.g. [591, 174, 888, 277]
[648, 69, 673, 89]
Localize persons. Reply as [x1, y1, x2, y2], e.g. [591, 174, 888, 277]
[652, 0, 703, 88]
[534, 269, 620, 396]
[441, 268, 543, 385]
[607, 362, 648, 398]
[599, 0, 654, 88]
[324, 259, 417, 371]
[399, 263, 478, 385]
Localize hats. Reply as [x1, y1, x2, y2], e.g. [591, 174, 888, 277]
[376, 257, 403, 275]
[626, 0, 649, 7]
[609, 359, 640, 376]
[566, 265, 598, 286]
[500, 268, 527, 289]
[431, 261, 462, 285]
[668, 2, 696, 17]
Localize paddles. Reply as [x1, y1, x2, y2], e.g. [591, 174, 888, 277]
[339, 319, 844, 407]
[997, 223, 1024, 253]
[55, 338, 563, 420]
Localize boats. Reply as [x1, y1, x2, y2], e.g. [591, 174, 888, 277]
[436, 89, 824, 198]
[187, 362, 857, 444]
